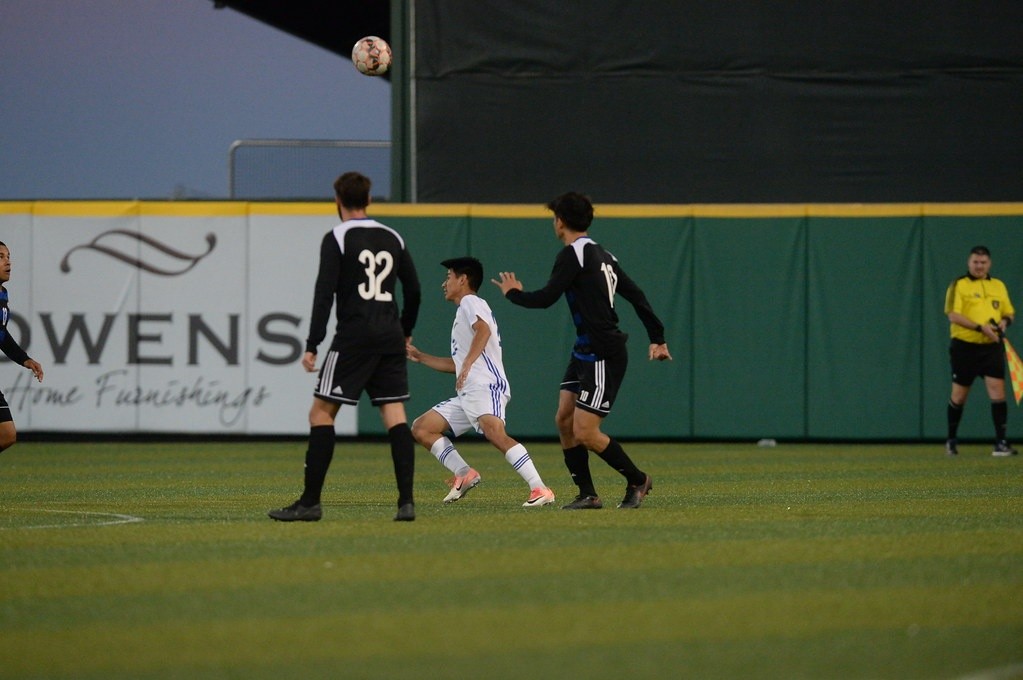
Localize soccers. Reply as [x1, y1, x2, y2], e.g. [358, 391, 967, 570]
[351, 35, 393, 77]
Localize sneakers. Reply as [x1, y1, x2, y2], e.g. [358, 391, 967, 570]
[393, 503, 415, 522]
[946, 440, 958, 455]
[267, 500, 322, 522]
[522, 487, 555, 507]
[562, 494, 602, 509]
[617, 475, 653, 508]
[991, 439, 1018, 456]
[442, 467, 481, 503]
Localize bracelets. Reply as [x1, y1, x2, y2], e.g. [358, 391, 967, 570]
[976, 325, 982, 332]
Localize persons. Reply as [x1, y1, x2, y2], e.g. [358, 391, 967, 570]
[944, 246, 1018, 456]
[491, 194, 672, 509]
[0, 241, 44, 452]
[267, 173, 422, 522]
[407, 257, 555, 506]
